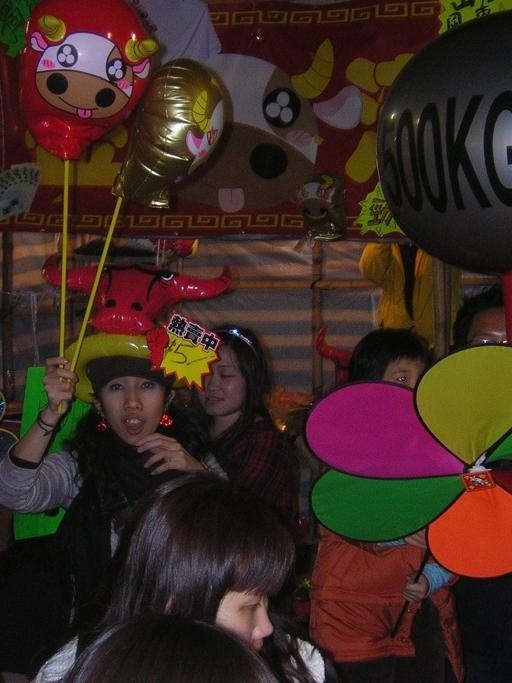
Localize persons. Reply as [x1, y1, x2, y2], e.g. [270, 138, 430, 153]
[62, 616, 276, 682]
[0, 353, 223, 632]
[34, 471, 326, 683]
[360, 241, 455, 360]
[179, 321, 303, 532]
[305, 327, 468, 683]
[451, 286, 510, 353]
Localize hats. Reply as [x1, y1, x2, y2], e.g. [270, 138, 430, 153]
[92, 355, 165, 391]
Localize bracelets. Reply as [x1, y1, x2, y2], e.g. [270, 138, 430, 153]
[39, 410, 57, 429]
[36, 419, 55, 437]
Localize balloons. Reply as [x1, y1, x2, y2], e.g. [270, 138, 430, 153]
[18, 0, 157, 163]
[375, 10, 512, 276]
[114, 58, 226, 211]
